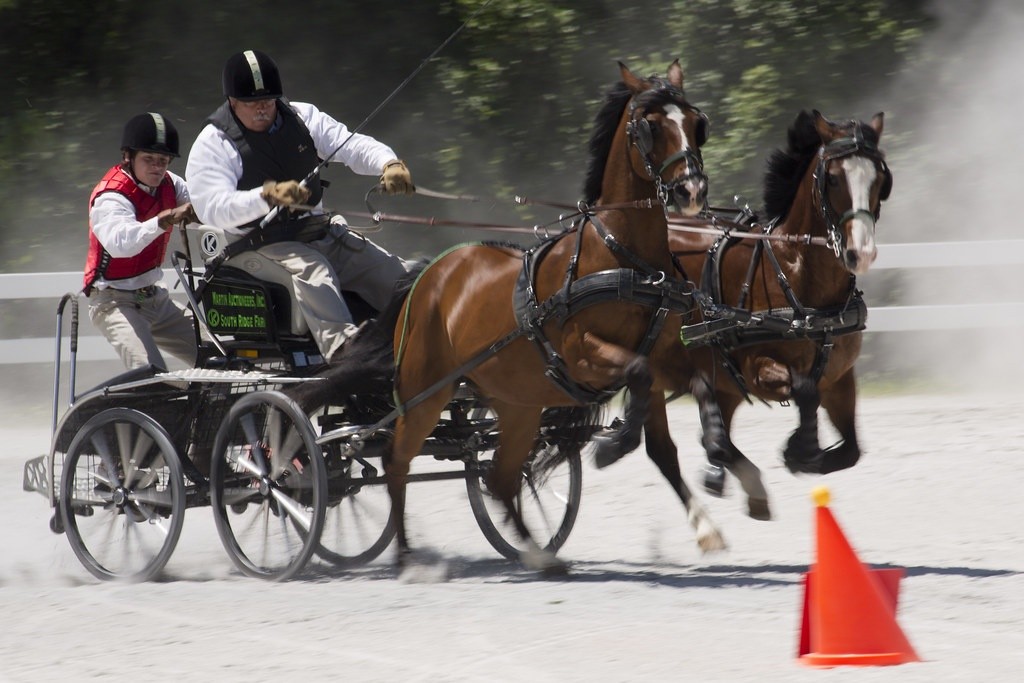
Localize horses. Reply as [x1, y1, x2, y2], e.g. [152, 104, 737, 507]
[388, 55, 714, 584]
[594, 103, 895, 557]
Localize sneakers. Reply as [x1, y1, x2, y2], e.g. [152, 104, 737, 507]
[188, 445, 251, 487]
[98, 456, 147, 482]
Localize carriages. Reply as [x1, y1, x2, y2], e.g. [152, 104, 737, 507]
[22, 57, 894, 584]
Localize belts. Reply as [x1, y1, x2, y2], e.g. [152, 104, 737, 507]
[91, 285, 155, 292]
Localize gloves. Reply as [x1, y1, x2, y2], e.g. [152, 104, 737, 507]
[380, 159, 413, 199]
[158, 203, 203, 230]
[263, 180, 312, 213]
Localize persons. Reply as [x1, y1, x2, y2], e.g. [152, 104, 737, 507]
[83, 111, 204, 372]
[185, 51, 420, 367]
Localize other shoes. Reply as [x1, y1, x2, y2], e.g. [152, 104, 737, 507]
[339, 318, 378, 353]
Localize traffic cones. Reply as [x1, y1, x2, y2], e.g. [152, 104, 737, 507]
[802, 488, 920, 666]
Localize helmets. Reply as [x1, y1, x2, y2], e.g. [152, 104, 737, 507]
[224, 50, 282, 100]
[120, 112, 181, 158]
